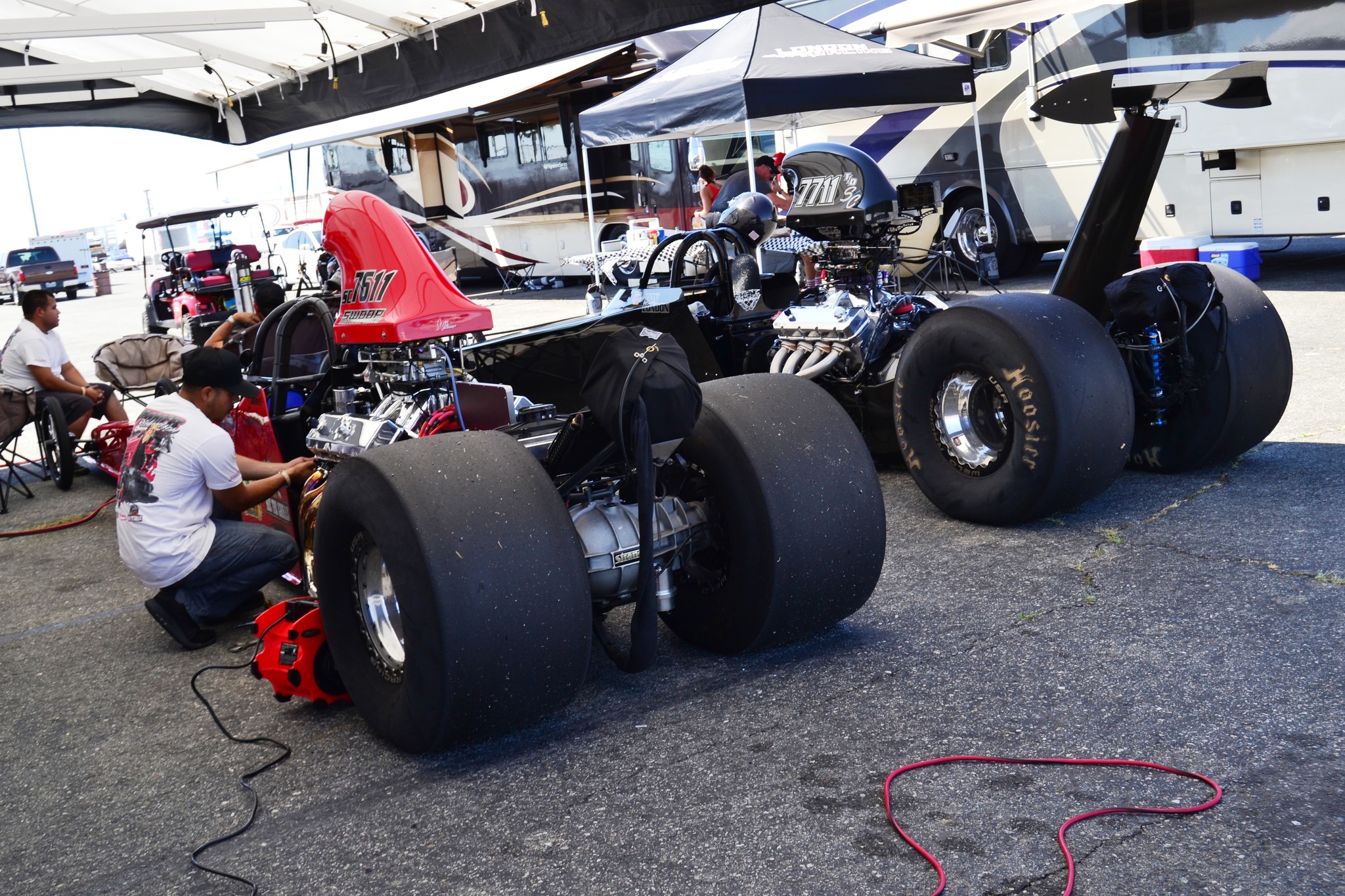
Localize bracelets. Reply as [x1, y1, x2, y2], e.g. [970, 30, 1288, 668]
[86, 385, 92, 388]
[699, 211, 701, 217]
[277, 470, 291, 487]
[80, 386, 86, 395]
[225, 314, 239, 328]
[778, 189, 783, 194]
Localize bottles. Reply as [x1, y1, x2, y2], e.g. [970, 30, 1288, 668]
[641, 227, 665, 246]
[674, 227, 681, 235]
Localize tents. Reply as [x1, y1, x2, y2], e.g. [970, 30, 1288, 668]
[580, 1, 993, 296]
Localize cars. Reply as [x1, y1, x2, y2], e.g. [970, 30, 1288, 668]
[102, 252, 136, 272]
[273, 224, 323, 291]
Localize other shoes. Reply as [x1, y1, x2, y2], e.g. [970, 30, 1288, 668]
[73, 463, 89, 476]
[144, 586, 216, 650]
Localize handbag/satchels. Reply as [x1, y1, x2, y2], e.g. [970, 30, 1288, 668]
[0, 393, 33, 438]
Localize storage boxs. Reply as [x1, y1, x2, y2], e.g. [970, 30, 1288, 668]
[601, 239, 622, 252]
[1140, 235, 1263, 280]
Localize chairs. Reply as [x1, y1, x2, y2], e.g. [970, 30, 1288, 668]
[879, 201, 969, 301]
[93, 334, 202, 408]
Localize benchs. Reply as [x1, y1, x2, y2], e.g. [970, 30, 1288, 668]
[181, 244, 275, 288]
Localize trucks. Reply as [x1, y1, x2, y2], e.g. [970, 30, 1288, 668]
[28, 232, 94, 290]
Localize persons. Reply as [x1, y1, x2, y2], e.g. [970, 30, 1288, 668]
[705, 155, 793, 233]
[963, 132, 1008, 199]
[114, 347, 322, 651]
[770, 152, 824, 298]
[0, 289, 130, 476]
[692, 164, 723, 218]
[200, 279, 286, 349]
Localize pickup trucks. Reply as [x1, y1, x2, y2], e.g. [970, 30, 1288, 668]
[0, 246, 79, 306]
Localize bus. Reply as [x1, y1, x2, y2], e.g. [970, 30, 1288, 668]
[204, 39, 775, 285]
[774, 0, 1345, 280]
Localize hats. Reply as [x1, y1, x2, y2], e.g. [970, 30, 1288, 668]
[180, 346, 261, 398]
[754, 155, 781, 175]
[251, 282, 285, 313]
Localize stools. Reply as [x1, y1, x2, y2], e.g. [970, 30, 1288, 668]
[496, 263, 539, 294]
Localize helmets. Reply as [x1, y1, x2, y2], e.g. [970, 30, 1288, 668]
[718, 192, 777, 246]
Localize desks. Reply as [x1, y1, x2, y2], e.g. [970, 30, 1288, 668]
[564, 244, 711, 317]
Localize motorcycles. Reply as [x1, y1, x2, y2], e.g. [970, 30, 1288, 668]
[136, 203, 282, 346]
